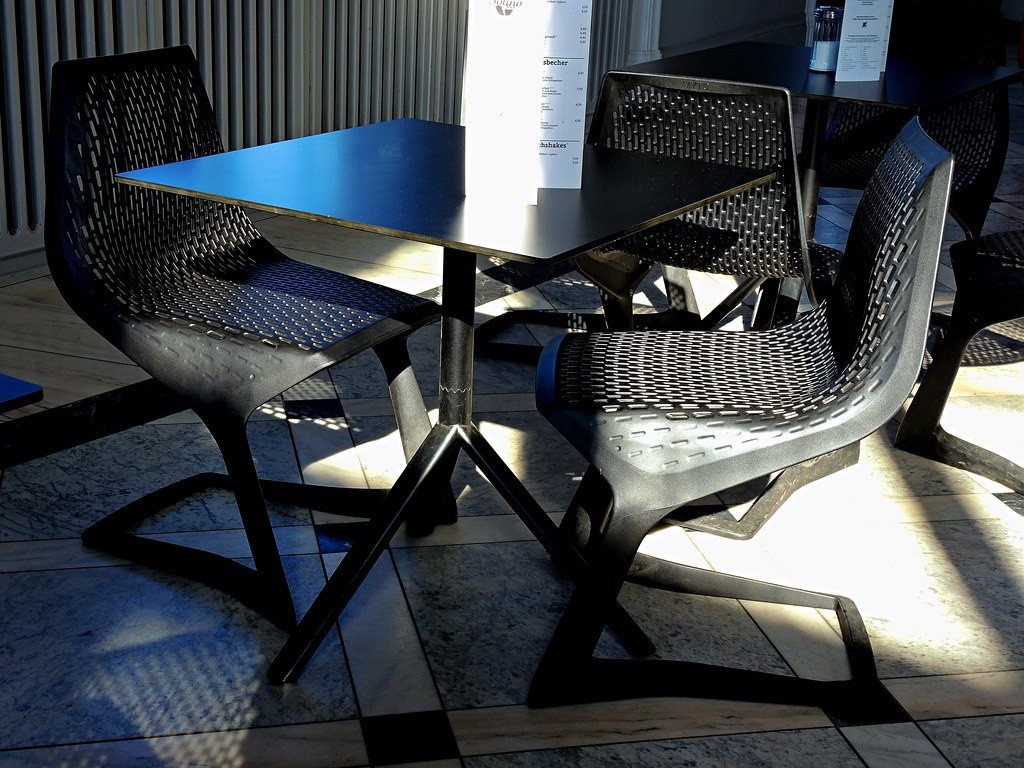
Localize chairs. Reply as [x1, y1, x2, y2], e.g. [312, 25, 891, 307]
[892, 228, 1024, 496]
[570, 70, 863, 539]
[762, 82, 1010, 328]
[525, 115, 955, 709]
[474, 256, 701, 362]
[44, 43, 459, 629]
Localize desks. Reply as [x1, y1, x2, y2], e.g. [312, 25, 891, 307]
[624, 40, 1024, 367]
[114, 118, 777, 683]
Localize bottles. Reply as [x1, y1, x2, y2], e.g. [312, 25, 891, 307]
[809, 7, 842, 73]
